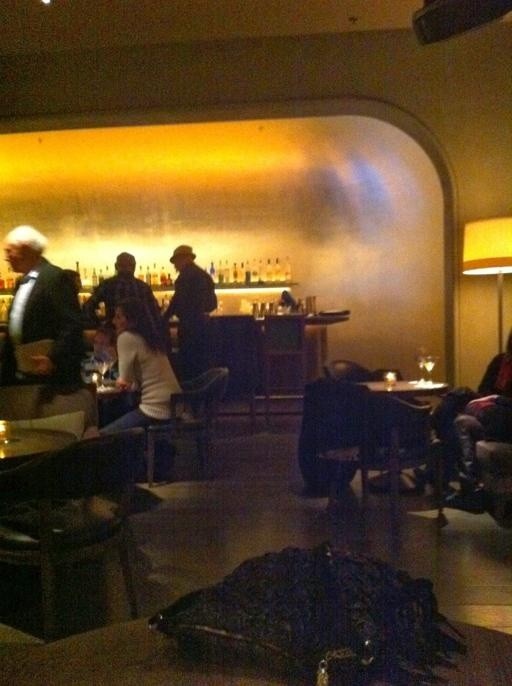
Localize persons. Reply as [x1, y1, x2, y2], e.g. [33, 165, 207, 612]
[413, 326, 512, 514]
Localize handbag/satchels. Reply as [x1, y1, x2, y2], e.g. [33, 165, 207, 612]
[148, 545, 465, 686]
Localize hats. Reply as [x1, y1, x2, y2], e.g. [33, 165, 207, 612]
[170, 246, 196, 264]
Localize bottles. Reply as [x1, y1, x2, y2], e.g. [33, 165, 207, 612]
[74, 256, 292, 282]
[216, 300, 224, 316]
[0, 267, 14, 321]
[78, 293, 107, 321]
[252, 296, 318, 319]
[158, 293, 179, 322]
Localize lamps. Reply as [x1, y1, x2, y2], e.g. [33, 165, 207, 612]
[461, 215, 511, 351]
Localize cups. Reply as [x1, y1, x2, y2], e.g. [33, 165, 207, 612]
[0, 419, 13, 445]
[384, 372, 398, 388]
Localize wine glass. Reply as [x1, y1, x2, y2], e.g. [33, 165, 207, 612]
[417, 352, 435, 383]
[82, 359, 115, 390]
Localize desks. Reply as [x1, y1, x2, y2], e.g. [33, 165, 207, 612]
[1, 426, 76, 461]
[84, 375, 141, 430]
[357, 380, 450, 494]
[0, 615, 512, 684]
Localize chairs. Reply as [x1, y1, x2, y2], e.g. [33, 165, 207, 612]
[316, 386, 449, 560]
[0, 424, 147, 640]
[144, 365, 232, 487]
[261, 312, 309, 400]
[475, 441, 511, 526]
[322, 358, 371, 386]
